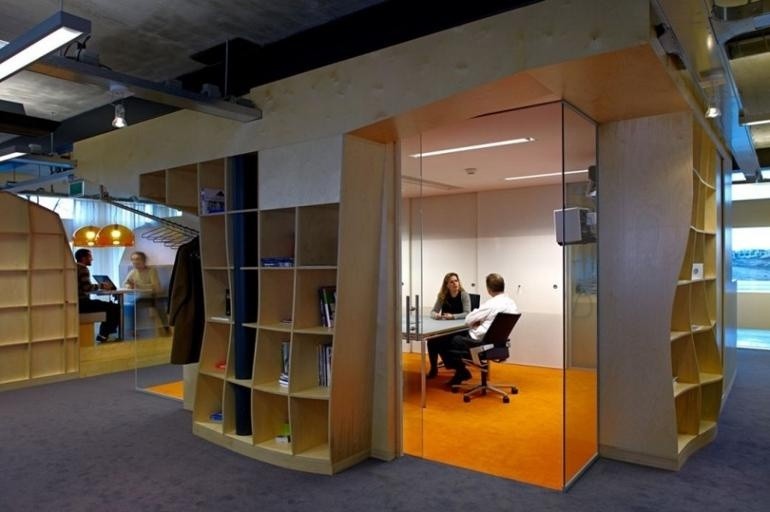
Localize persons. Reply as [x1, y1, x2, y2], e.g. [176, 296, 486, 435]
[124, 251, 172, 337]
[446, 272, 518, 385]
[425, 272, 473, 381]
[74, 248, 122, 343]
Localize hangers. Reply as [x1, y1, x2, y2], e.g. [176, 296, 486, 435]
[140, 217, 198, 253]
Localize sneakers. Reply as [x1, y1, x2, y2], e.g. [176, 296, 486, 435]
[96, 335, 112, 342]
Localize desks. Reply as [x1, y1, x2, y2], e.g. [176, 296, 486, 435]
[402, 315, 468, 408]
[87, 288, 152, 341]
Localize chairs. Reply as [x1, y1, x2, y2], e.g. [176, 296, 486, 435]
[437, 293, 521, 404]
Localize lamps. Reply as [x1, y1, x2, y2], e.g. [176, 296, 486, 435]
[705, 79, 721, 118]
[72, 200, 136, 247]
[106, 89, 128, 128]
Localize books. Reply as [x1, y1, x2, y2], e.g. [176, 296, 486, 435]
[275, 435, 290, 443]
[279, 318, 292, 326]
[318, 285, 327, 327]
[210, 313, 230, 322]
[209, 411, 223, 424]
[262, 254, 293, 268]
[199, 185, 226, 215]
[278, 340, 332, 393]
[214, 360, 226, 370]
[323, 286, 336, 327]
[225, 287, 232, 316]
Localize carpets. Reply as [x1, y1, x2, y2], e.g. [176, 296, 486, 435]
[137, 352, 598, 493]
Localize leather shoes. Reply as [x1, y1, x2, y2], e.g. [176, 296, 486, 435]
[429, 369, 437, 377]
[451, 370, 470, 385]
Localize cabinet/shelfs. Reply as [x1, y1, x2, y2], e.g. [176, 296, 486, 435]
[597, 110, 724, 473]
[138, 134, 387, 476]
